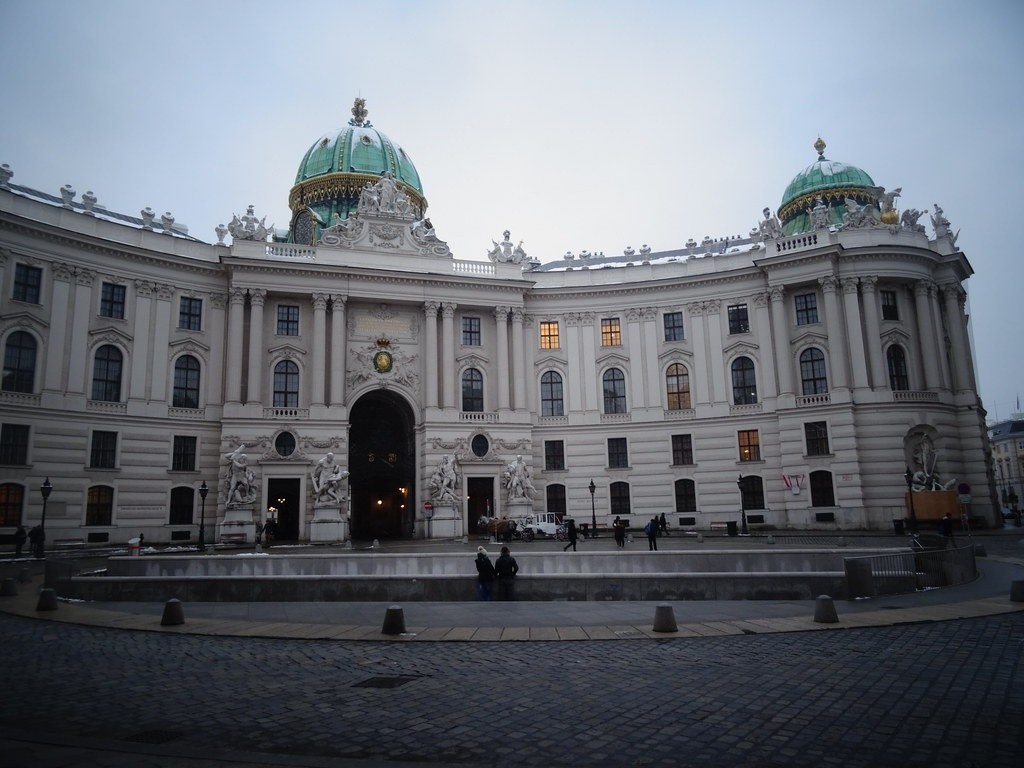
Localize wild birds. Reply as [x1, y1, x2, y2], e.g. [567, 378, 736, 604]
[866, 182, 903, 212]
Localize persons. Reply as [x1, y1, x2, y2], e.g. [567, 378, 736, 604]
[613, 516, 625, 547]
[14, 525, 45, 557]
[901, 209, 925, 232]
[564, 519, 577, 551]
[475, 546, 519, 601]
[359, 172, 411, 215]
[429, 455, 459, 501]
[502, 516, 511, 542]
[837, 205, 877, 231]
[932, 204, 944, 225]
[224, 450, 257, 508]
[261, 519, 277, 542]
[504, 455, 537, 502]
[942, 513, 960, 554]
[413, 220, 447, 244]
[644, 513, 670, 551]
[312, 452, 349, 505]
[320, 212, 360, 232]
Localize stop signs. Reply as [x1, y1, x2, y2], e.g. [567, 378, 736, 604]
[425, 502, 431, 509]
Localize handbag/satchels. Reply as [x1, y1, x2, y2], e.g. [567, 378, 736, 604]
[644, 522, 651, 534]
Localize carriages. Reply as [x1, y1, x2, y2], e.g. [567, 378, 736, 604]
[477, 510, 566, 544]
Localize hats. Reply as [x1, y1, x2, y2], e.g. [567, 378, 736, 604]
[500, 547, 510, 553]
[478, 546, 488, 554]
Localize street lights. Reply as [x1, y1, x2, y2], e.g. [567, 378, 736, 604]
[198, 480, 208, 550]
[41, 477, 53, 554]
[904, 465, 919, 534]
[737, 474, 748, 534]
[588, 479, 599, 537]
